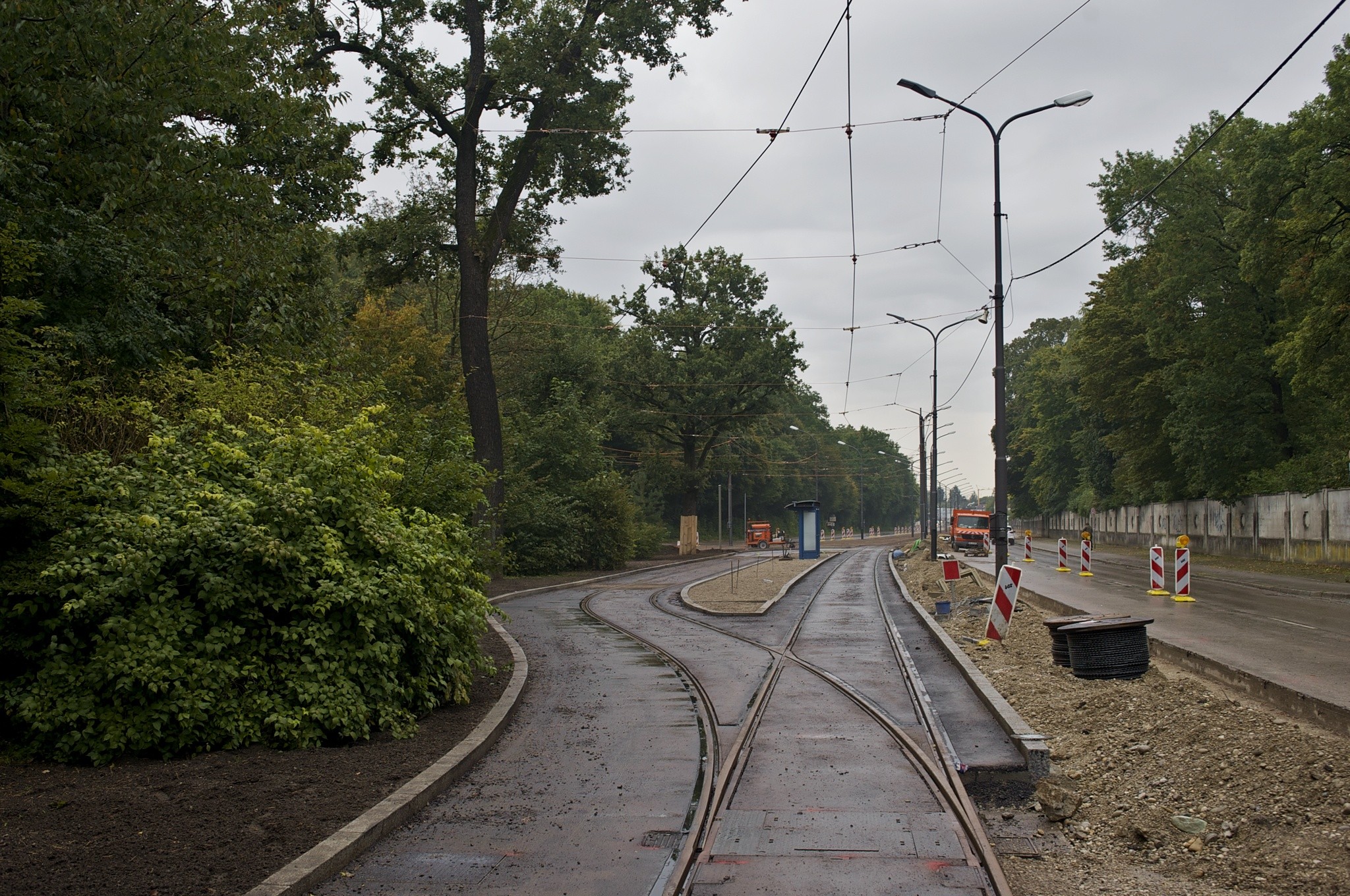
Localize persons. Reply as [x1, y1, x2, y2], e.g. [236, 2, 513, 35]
[1081, 522, 1093, 551]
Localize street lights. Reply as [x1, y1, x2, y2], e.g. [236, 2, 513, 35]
[790, 425, 818, 501]
[895, 78, 1095, 587]
[939, 472, 975, 533]
[880, 450, 946, 538]
[838, 440, 864, 540]
[887, 308, 986, 563]
[917, 406, 953, 535]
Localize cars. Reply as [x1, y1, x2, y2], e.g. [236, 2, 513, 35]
[1007, 525, 1016, 546]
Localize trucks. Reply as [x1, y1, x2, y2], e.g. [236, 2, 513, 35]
[950, 510, 991, 552]
[745, 521, 799, 550]
